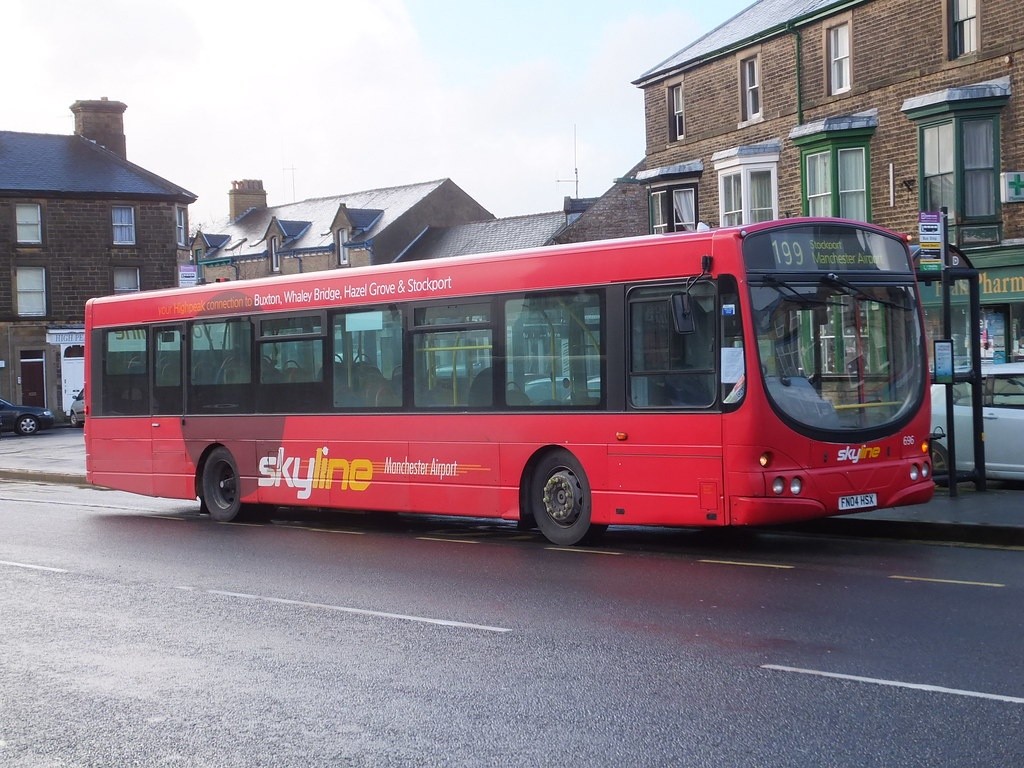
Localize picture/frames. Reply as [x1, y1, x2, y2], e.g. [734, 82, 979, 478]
[933, 339, 955, 384]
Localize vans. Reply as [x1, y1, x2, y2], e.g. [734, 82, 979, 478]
[434, 362, 487, 391]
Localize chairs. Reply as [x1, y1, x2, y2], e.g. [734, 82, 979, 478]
[128, 350, 528, 409]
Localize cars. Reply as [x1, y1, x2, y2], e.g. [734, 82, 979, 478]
[523, 377, 600, 399]
[930, 361, 1024, 485]
[0, 399, 55, 436]
[69, 389, 85, 427]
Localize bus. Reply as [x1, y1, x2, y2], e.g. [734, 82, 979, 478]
[83, 217, 935, 546]
